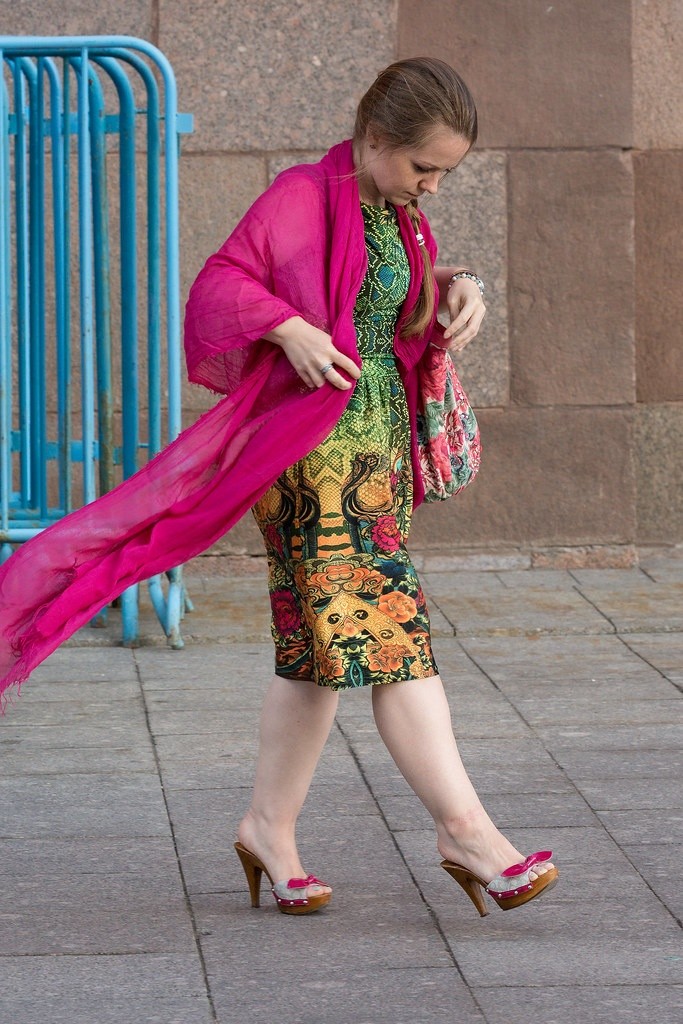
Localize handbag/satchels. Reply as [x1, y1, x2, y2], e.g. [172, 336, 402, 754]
[423, 349, 483, 504]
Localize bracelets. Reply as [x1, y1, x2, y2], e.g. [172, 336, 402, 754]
[448, 273, 484, 296]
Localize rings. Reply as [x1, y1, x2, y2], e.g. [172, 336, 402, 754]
[320, 364, 333, 376]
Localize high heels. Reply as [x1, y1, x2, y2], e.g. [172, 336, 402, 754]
[234, 841, 332, 915]
[440, 851, 559, 916]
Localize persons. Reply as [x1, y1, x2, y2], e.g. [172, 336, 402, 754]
[183, 57, 558, 918]
